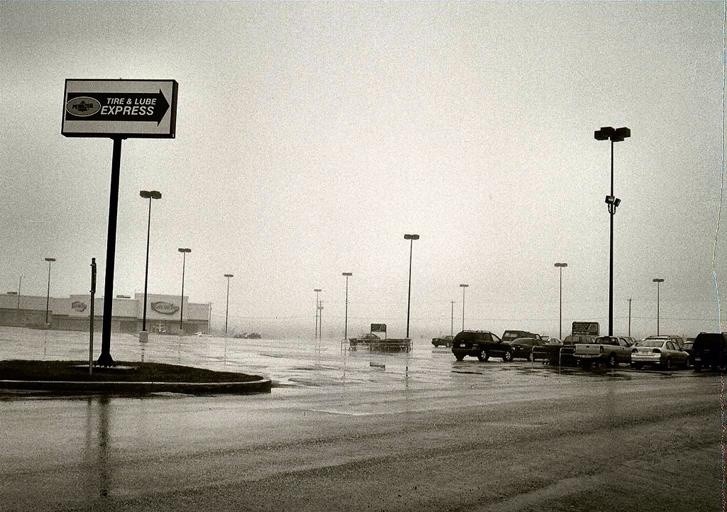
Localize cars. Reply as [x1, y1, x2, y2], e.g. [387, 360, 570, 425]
[631, 339, 690, 371]
[232, 331, 263, 341]
[545, 338, 563, 345]
[507, 337, 544, 362]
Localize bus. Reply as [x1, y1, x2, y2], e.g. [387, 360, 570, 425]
[503, 331, 542, 346]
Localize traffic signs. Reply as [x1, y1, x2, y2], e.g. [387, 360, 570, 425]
[61, 77, 177, 142]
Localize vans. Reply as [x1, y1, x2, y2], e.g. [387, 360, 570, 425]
[645, 336, 685, 349]
[692, 332, 727, 372]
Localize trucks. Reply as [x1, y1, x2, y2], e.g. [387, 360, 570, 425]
[543, 333, 603, 365]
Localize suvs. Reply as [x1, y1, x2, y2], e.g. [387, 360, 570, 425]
[450, 331, 513, 362]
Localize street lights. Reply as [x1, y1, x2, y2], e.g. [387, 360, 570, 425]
[342, 272, 353, 342]
[177, 247, 192, 332]
[312, 288, 324, 339]
[224, 274, 235, 335]
[626, 298, 632, 337]
[595, 126, 632, 337]
[652, 278, 665, 336]
[42, 257, 59, 330]
[138, 190, 162, 332]
[460, 282, 469, 331]
[553, 261, 570, 341]
[450, 299, 456, 334]
[403, 234, 420, 341]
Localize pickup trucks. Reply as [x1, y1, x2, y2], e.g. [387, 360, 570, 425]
[432, 335, 454, 348]
[573, 336, 638, 368]
